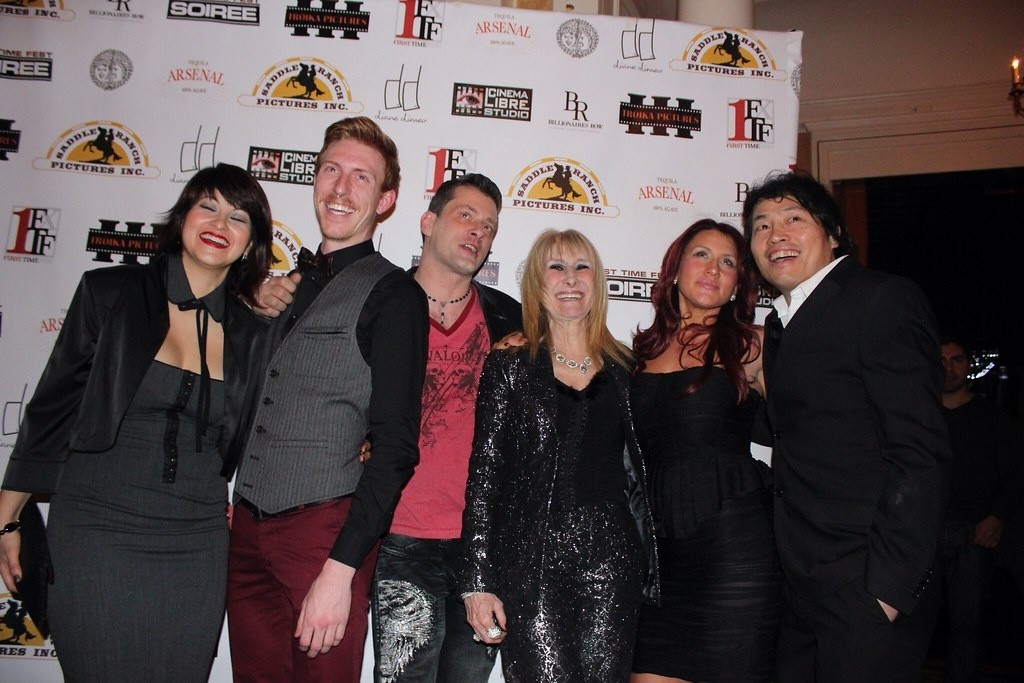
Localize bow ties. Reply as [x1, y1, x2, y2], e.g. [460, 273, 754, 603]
[297, 247, 339, 280]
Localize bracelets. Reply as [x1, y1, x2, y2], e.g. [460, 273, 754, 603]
[0, 522, 23, 536]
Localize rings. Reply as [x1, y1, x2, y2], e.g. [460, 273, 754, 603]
[485, 625, 502, 638]
[472, 634, 484, 643]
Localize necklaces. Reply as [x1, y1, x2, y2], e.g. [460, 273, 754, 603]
[420, 288, 473, 331]
[547, 343, 594, 378]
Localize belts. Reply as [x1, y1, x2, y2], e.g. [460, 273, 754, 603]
[239, 493, 354, 520]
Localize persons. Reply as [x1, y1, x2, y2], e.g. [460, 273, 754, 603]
[742, 170, 946, 683]
[459, 227, 664, 683]
[0, 162, 371, 683]
[223, 117, 432, 683]
[928, 329, 1016, 683]
[487, 216, 781, 683]
[368, 172, 523, 683]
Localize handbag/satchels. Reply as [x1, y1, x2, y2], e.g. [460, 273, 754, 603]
[0, 501, 54, 640]
